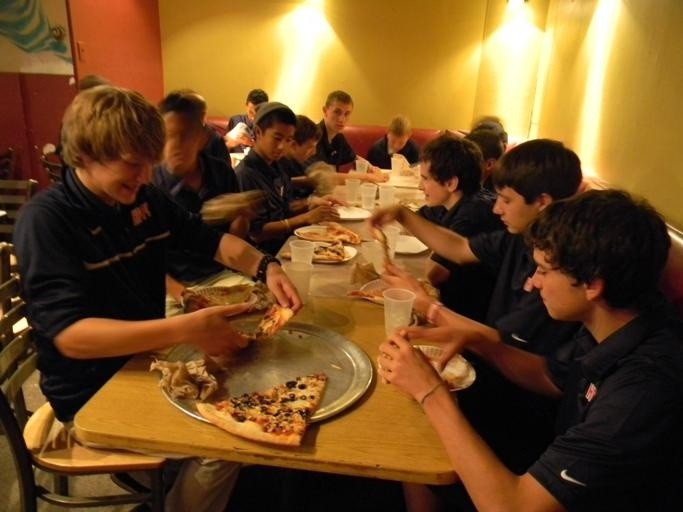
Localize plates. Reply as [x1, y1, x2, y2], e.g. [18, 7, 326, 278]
[204, 275, 257, 285]
[376, 343, 482, 392]
[394, 232, 431, 257]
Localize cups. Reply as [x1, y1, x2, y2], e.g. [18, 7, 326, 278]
[282, 157, 416, 340]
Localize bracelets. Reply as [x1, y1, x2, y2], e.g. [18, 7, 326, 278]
[258, 255, 282, 281]
[419, 378, 445, 405]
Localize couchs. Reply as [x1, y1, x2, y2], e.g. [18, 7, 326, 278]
[203, 117, 683, 347]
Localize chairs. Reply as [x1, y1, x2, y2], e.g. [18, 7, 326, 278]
[0, 147, 23, 225]
[0, 179, 39, 233]
[0, 242, 169, 512]
[40, 154, 65, 182]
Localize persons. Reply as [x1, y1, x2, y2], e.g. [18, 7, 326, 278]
[158, 89, 255, 315]
[226, 89, 389, 257]
[378, 190, 683, 512]
[369, 137, 582, 360]
[411, 117, 508, 315]
[365, 116, 419, 169]
[12, 84, 302, 511]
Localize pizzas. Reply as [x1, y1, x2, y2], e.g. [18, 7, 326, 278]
[372, 225, 390, 267]
[232, 303, 293, 342]
[348, 281, 434, 302]
[197, 373, 329, 446]
[297, 221, 361, 244]
[181, 284, 264, 316]
[312, 242, 350, 262]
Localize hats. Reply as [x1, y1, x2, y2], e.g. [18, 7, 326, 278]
[252, 101, 296, 128]
